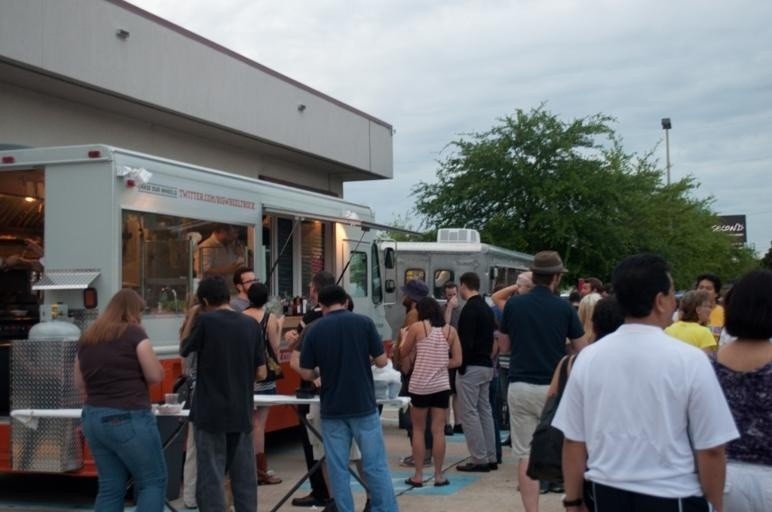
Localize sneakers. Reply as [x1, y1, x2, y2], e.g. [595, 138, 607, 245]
[456, 463, 490, 472]
[399, 456, 429, 467]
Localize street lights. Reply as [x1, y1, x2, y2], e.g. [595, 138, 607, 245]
[661, 118, 672, 184]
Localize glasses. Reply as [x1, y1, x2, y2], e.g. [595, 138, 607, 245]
[241, 278, 259, 285]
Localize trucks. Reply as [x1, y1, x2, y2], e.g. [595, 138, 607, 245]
[381, 227, 535, 344]
[0, 142, 398, 500]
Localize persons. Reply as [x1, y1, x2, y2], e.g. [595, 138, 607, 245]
[440, 282, 462, 435]
[179, 277, 267, 512]
[581, 278, 602, 296]
[484, 283, 507, 319]
[194, 225, 246, 284]
[550, 253, 740, 511]
[569, 292, 582, 310]
[290, 293, 372, 512]
[500, 250, 590, 512]
[74, 288, 167, 512]
[662, 290, 718, 353]
[392, 281, 433, 468]
[241, 282, 285, 485]
[399, 297, 462, 487]
[229, 267, 257, 314]
[705, 271, 772, 512]
[492, 272, 535, 312]
[545, 298, 624, 400]
[577, 292, 601, 339]
[284, 272, 335, 506]
[696, 275, 725, 341]
[491, 361, 502, 464]
[179, 295, 198, 508]
[300, 285, 398, 512]
[455, 272, 498, 473]
[491, 331, 511, 446]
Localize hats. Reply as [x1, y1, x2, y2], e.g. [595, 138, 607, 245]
[529, 250, 569, 274]
[399, 278, 430, 302]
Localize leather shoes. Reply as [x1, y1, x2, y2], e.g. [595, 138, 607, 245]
[292, 494, 329, 507]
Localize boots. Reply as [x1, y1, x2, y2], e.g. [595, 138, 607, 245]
[256, 451, 282, 486]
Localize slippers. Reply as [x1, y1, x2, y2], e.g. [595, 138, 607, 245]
[404, 476, 424, 488]
[433, 478, 451, 486]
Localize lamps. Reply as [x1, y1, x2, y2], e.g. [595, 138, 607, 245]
[24, 181, 37, 203]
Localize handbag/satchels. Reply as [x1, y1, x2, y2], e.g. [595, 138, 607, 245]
[526, 352, 573, 481]
[256, 310, 284, 383]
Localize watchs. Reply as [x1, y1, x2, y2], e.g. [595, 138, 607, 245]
[561, 495, 584, 508]
